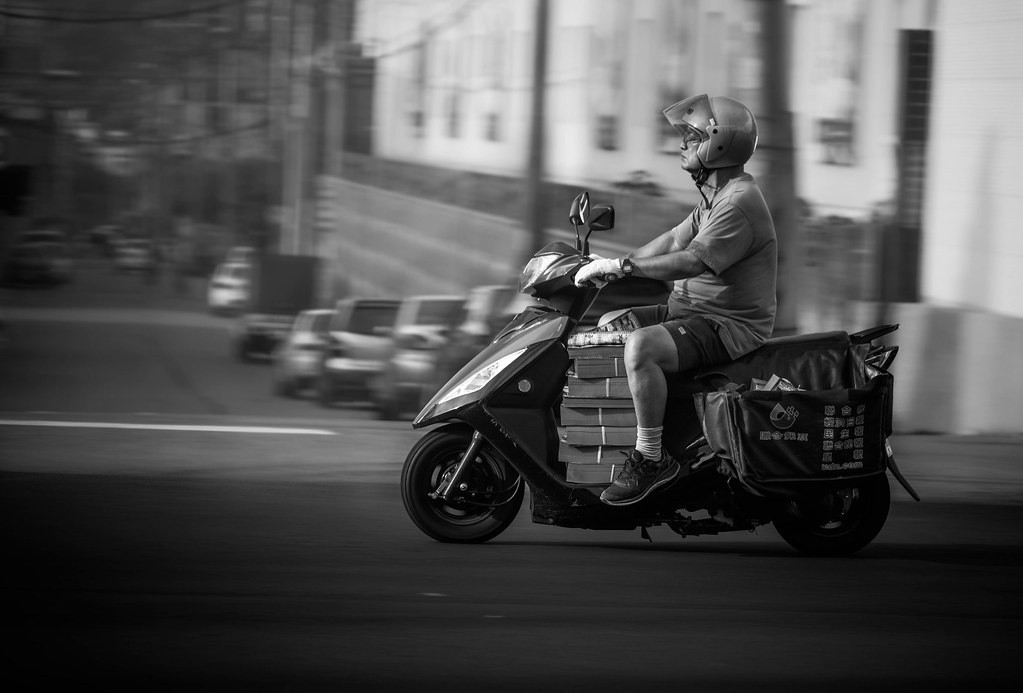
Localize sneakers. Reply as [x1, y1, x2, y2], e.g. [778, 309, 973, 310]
[597, 448, 681, 507]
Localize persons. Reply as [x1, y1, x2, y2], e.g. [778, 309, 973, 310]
[572, 95, 777, 506]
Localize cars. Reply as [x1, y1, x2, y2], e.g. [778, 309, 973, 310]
[11, 217, 525, 421]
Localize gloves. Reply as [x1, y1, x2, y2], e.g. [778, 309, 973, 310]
[571, 250, 624, 292]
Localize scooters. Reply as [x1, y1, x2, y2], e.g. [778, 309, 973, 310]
[397, 193, 919, 556]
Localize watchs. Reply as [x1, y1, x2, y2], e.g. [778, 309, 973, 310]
[621, 257, 634, 280]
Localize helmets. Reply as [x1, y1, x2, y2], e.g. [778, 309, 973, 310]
[661, 94, 759, 170]
